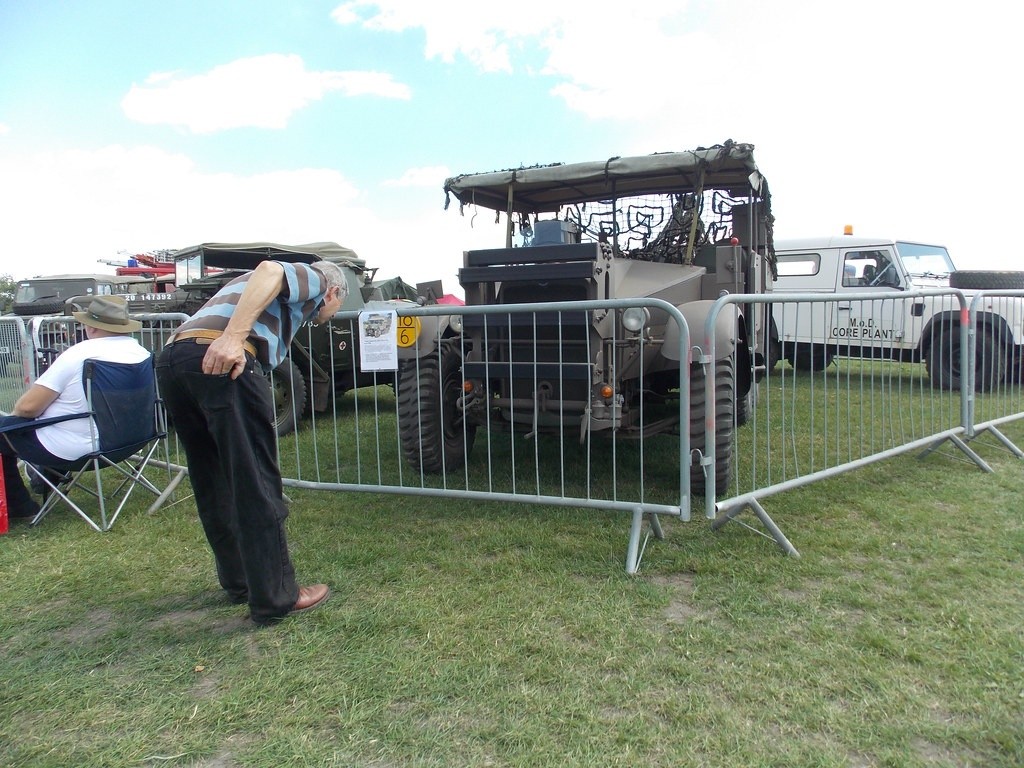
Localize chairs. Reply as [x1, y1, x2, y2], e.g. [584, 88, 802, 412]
[0, 350, 175, 533]
[863, 265, 886, 285]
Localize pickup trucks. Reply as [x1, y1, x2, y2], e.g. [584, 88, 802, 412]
[769, 236, 1024, 392]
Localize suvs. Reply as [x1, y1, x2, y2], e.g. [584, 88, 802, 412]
[159, 240, 459, 438]
[397, 138, 779, 497]
[11, 275, 157, 359]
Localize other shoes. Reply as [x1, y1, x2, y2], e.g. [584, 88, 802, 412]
[25, 464, 41, 493]
[8, 501, 41, 517]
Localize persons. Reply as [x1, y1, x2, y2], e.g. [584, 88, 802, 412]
[0, 295, 156, 523]
[155, 260, 349, 629]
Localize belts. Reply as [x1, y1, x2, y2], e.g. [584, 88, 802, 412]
[165, 329, 258, 358]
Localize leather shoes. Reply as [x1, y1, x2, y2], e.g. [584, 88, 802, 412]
[287, 584, 331, 613]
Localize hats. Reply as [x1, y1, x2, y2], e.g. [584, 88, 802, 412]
[72, 295, 142, 333]
